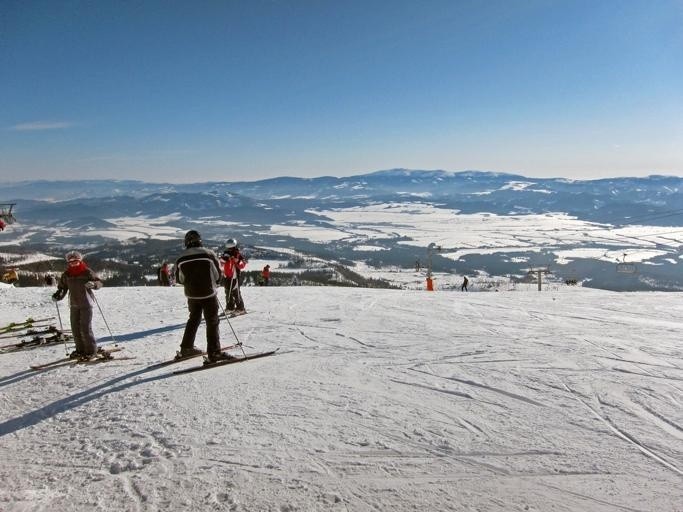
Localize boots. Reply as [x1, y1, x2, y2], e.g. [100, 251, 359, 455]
[208, 351, 233, 360]
[70, 351, 97, 360]
[181, 347, 202, 355]
[222, 309, 246, 317]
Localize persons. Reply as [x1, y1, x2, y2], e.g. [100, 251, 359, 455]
[174, 230, 234, 361]
[160, 259, 171, 288]
[219, 249, 244, 315]
[221, 237, 246, 313]
[461, 275, 467, 290]
[51, 251, 102, 360]
[262, 264, 270, 286]
[44, 274, 51, 287]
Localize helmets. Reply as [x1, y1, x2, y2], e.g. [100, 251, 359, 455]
[225, 238, 237, 248]
[185, 231, 201, 243]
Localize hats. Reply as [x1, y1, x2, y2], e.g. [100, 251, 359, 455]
[65, 251, 81, 262]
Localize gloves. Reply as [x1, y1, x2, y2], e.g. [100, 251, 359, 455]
[84, 281, 95, 289]
[52, 290, 64, 301]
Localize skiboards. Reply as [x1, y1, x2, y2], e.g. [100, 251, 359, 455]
[148, 342, 280, 375]
[0, 317, 74, 354]
[30, 347, 137, 371]
[200, 308, 256, 323]
